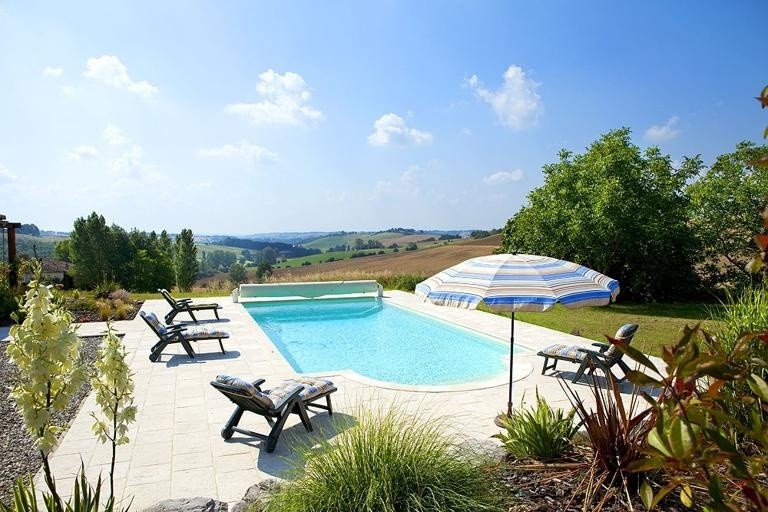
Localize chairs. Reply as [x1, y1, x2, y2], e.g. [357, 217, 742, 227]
[536, 323, 639, 384]
[210, 374, 338, 454]
[138, 287, 230, 362]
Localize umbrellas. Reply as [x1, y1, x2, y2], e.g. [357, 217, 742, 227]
[414, 253, 621, 418]
